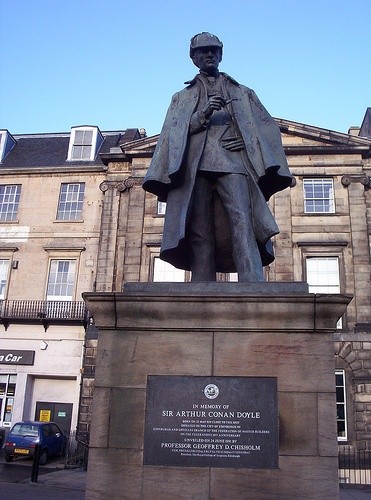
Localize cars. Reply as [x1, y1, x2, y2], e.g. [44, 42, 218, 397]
[2, 421, 69, 465]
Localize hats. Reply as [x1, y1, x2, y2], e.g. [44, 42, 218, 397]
[189, 31, 224, 59]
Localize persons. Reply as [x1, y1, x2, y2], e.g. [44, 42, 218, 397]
[142, 33, 293, 282]
